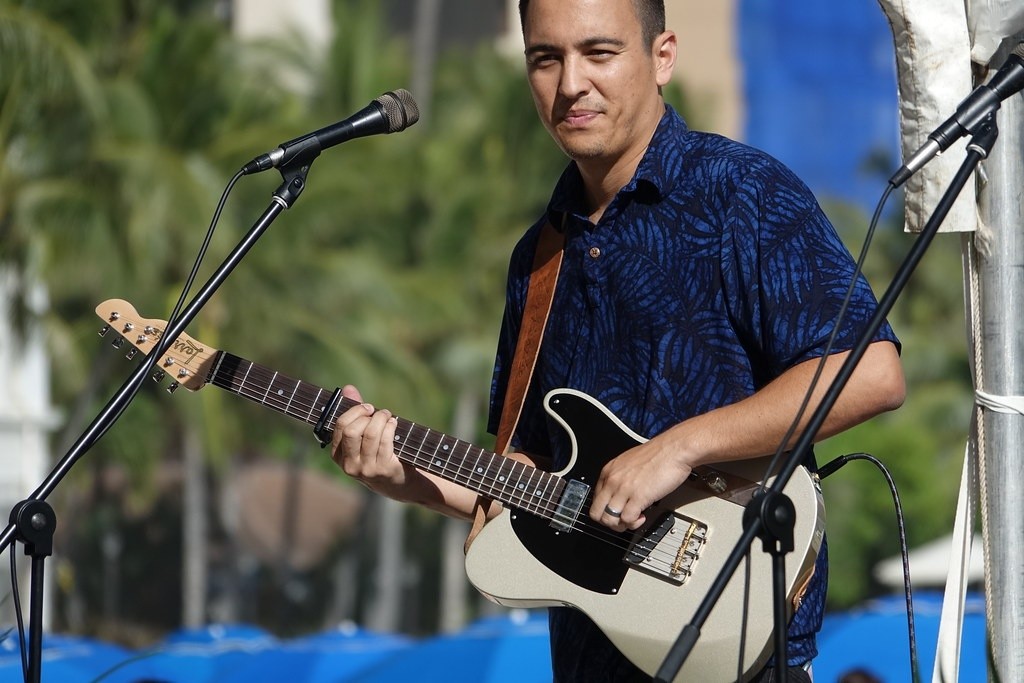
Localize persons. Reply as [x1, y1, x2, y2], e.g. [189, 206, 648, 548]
[332, 0, 906, 682]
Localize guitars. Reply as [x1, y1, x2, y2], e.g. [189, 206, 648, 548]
[90, 296, 827, 683]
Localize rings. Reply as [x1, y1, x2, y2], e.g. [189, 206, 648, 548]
[605, 506, 622, 517]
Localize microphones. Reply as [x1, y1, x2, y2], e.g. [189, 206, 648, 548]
[889, 43, 1024, 188]
[243, 88, 420, 174]
